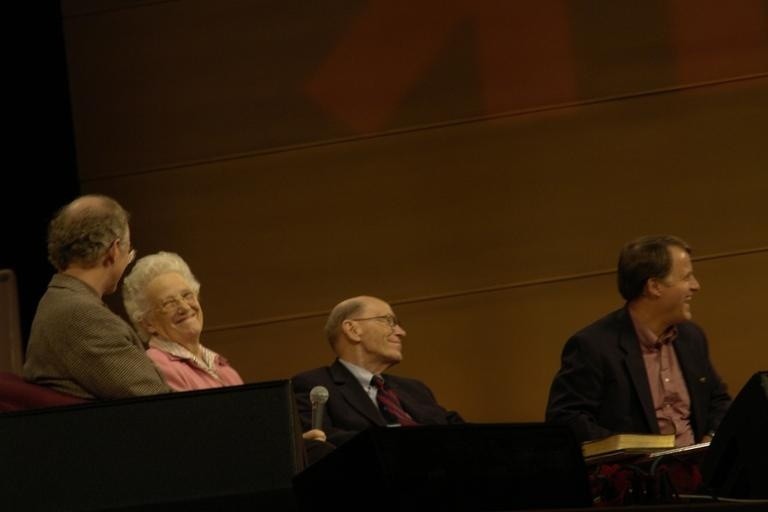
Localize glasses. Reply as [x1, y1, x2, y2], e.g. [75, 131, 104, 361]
[109, 230, 135, 263]
[353, 315, 402, 327]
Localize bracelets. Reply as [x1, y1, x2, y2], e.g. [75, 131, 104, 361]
[706, 431, 716, 438]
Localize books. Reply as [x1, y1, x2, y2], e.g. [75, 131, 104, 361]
[580, 434, 676, 458]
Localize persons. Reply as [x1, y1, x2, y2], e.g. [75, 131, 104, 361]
[22, 195, 172, 402]
[120, 251, 328, 443]
[545, 232, 733, 507]
[291, 296, 466, 449]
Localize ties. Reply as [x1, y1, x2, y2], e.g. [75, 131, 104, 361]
[371, 375, 421, 425]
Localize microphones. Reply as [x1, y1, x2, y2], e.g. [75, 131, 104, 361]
[309, 386, 329, 430]
